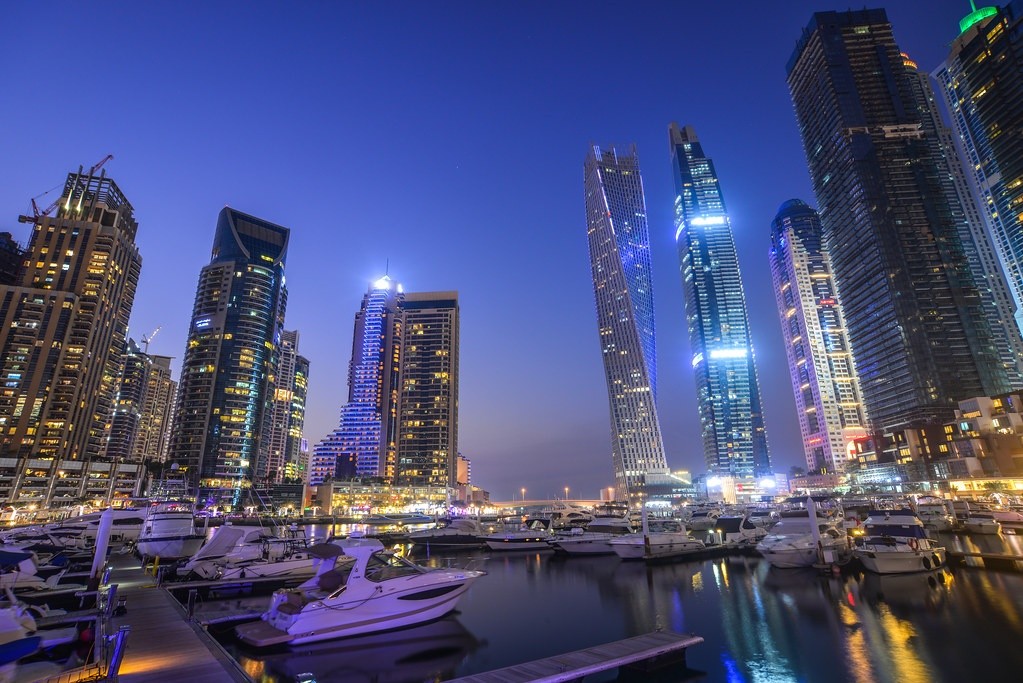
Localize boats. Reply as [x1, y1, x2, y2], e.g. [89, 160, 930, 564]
[261, 530, 486, 648]
[0, 478, 1023, 683]
[260, 614, 490, 682]
